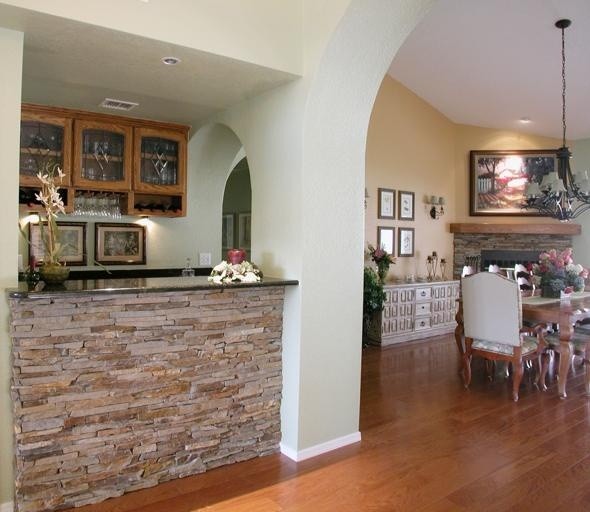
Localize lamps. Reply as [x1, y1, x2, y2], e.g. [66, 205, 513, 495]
[430, 195, 445, 219]
[524, 15, 590, 221]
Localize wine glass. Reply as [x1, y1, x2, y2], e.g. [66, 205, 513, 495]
[71, 190, 124, 218]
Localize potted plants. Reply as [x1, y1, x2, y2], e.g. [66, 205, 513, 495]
[18, 159, 112, 285]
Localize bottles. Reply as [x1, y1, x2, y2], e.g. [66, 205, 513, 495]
[27, 255, 39, 281]
[182, 258, 194, 277]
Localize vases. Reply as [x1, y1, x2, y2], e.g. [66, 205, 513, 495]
[537, 282, 563, 298]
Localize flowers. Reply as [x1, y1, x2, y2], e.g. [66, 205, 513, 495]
[530, 246, 588, 296]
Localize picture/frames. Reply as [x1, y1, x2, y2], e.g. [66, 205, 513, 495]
[469, 149, 564, 217]
[377, 226, 395, 257]
[378, 188, 395, 220]
[398, 227, 414, 257]
[28, 221, 88, 267]
[239, 211, 251, 253]
[398, 191, 415, 221]
[222, 212, 236, 251]
[95, 222, 146, 265]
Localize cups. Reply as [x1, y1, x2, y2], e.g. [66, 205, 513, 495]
[227, 250, 246, 264]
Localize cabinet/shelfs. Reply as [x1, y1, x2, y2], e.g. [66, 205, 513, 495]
[368, 286, 414, 347]
[414, 284, 431, 339]
[19, 102, 73, 214]
[72, 109, 132, 218]
[131, 118, 192, 217]
[432, 283, 459, 337]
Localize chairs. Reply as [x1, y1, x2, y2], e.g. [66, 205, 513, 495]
[455, 272, 551, 404]
[460, 260, 535, 293]
